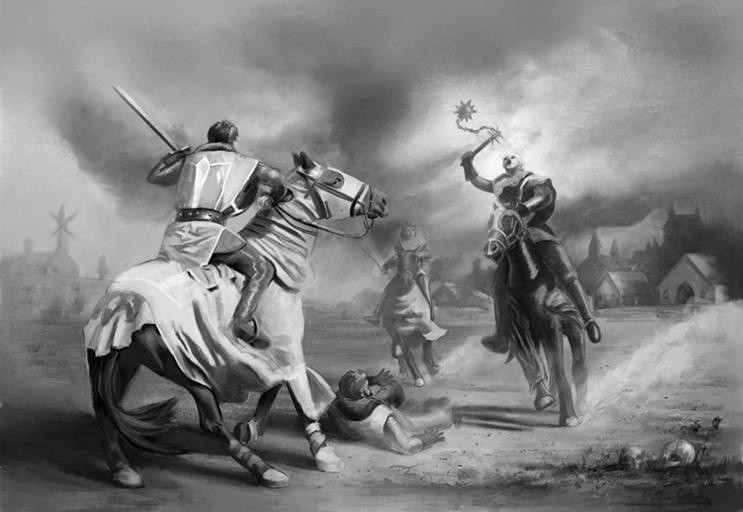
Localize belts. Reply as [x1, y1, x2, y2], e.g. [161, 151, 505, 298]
[175, 208, 226, 226]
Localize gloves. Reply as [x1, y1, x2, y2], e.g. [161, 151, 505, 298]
[514, 204, 529, 218]
[462, 151, 478, 181]
[411, 429, 446, 452]
[367, 368, 395, 387]
[160, 145, 194, 169]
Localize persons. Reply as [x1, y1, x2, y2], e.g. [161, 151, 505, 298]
[363, 222, 435, 326]
[317, 368, 459, 455]
[460, 150, 600, 354]
[147, 121, 287, 349]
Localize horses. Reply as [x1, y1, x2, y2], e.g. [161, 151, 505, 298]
[482, 195, 589, 428]
[380, 237, 440, 388]
[80, 151, 389, 488]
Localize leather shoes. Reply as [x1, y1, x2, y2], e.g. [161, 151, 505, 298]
[363, 316, 380, 326]
[233, 320, 270, 348]
[481, 335, 509, 353]
[431, 310, 434, 321]
[584, 316, 601, 343]
